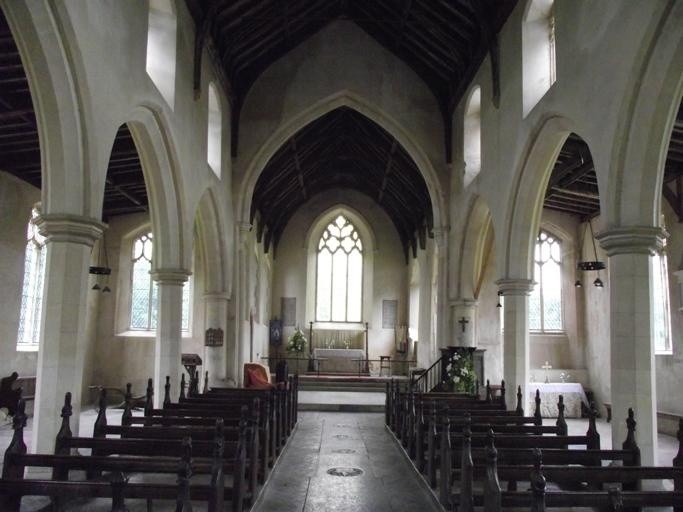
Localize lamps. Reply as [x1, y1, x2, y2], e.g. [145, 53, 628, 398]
[89, 231, 112, 292]
[574, 175, 607, 288]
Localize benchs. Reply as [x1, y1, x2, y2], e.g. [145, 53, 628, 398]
[386, 377, 683, 512]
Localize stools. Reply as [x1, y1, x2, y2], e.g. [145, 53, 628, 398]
[379, 355, 393, 378]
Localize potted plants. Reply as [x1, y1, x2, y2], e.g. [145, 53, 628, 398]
[286, 325, 309, 375]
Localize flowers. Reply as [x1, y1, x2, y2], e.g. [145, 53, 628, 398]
[442, 350, 479, 393]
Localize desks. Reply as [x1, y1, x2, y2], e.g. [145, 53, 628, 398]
[312, 348, 366, 374]
[351, 360, 416, 377]
[261, 356, 328, 376]
